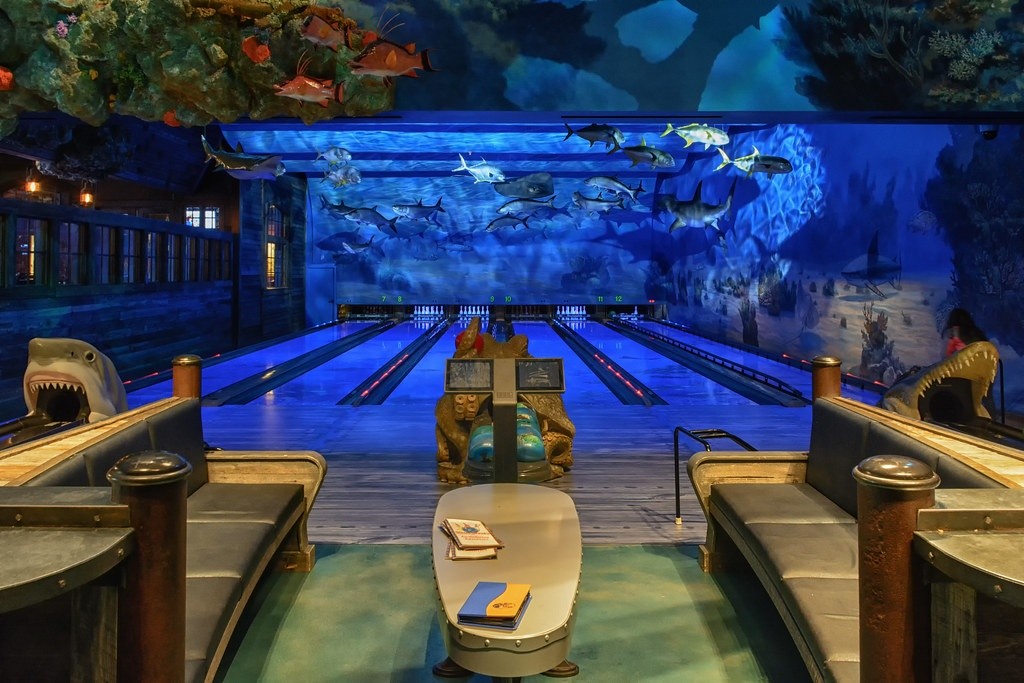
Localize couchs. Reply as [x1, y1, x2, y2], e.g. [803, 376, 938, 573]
[2, 352, 328, 682]
[687, 396, 1023, 683]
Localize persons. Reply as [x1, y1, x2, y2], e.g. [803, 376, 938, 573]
[939, 307, 986, 362]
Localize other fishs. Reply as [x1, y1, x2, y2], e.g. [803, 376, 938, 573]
[197, 12, 956, 299]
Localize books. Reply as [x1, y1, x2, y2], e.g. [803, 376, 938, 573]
[444, 537, 453, 559]
[455, 580, 534, 630]
[438, 521, 452, 536]
[450, 536, 499, 561]
[443, 517, 506, 549]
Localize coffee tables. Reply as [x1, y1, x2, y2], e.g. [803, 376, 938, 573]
[432, 483, 582, 682]
